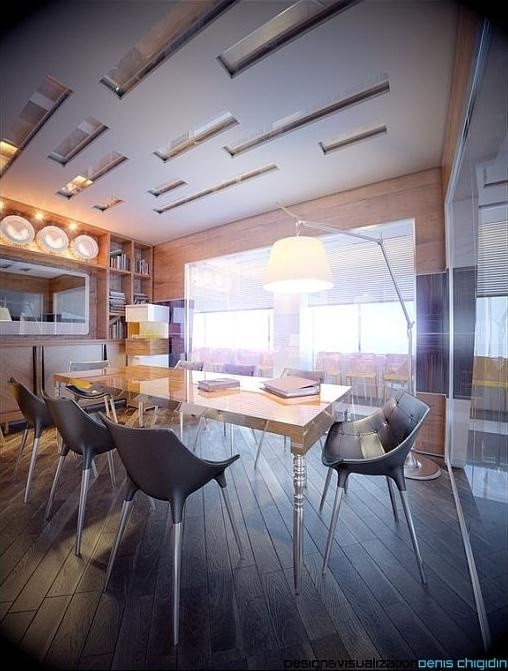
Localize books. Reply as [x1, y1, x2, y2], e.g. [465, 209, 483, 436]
[197, 377, 241, 393]
[108, 290, 127, 313]
[108, 316, 127, 339]
[133, 291, 149, 305]
[133, 257, 149, 275]
[110, 248, 129, 271]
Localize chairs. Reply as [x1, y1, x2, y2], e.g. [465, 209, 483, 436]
[8, 354, 430, 645]
[464, 356, 508, 505]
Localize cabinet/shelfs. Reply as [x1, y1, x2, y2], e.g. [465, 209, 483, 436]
[96, 233, 154, 342]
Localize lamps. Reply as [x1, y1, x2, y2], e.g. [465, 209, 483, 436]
[264, 218, 442, 482]
